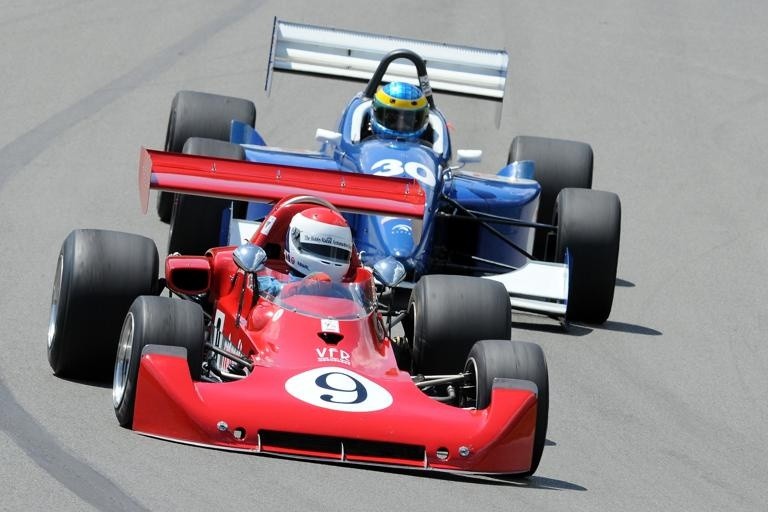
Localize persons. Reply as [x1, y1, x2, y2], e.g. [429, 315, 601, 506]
[262, 206, 373, 321]
[359, 79, 434, 145]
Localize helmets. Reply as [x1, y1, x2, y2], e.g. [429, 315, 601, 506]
[285, 208, 353, 282]
[369, 82, 430, 141]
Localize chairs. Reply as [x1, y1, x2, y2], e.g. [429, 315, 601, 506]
[241, 194, 375, 294]
[338, 45, 450, 159]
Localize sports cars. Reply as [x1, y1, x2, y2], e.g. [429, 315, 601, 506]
[42, 140, 552, 489]
[153, 15, 625, 330]
